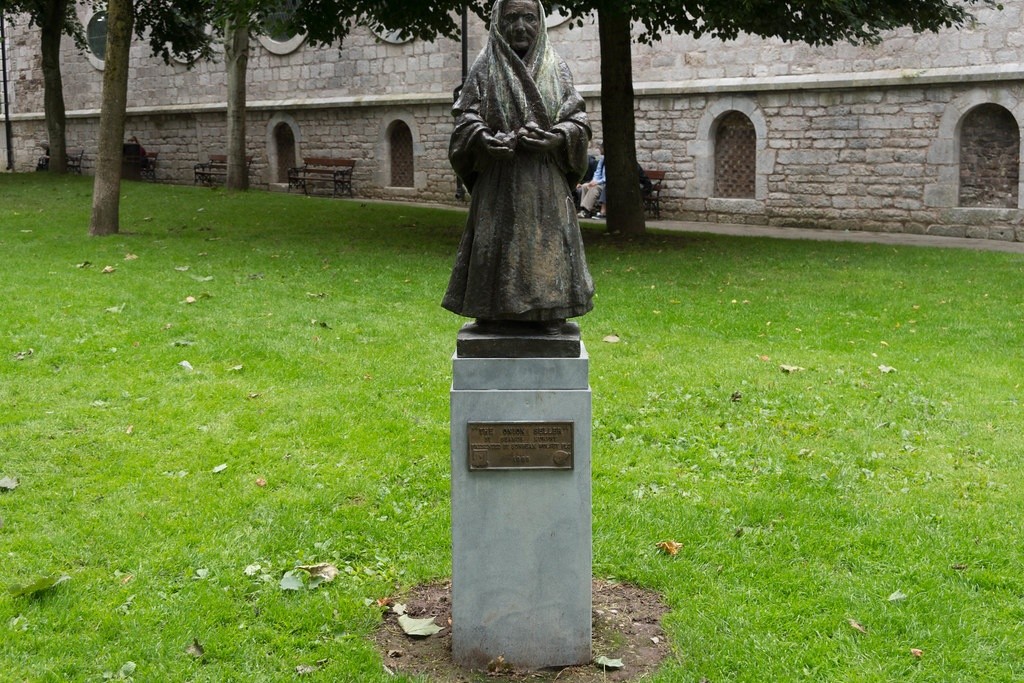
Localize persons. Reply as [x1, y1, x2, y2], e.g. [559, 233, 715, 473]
[570, 140, 651, 219]
[441, 0, 595, 326]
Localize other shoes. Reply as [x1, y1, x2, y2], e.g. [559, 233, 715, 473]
[577, 211, 586, 218]
[592, 213, 607, 220]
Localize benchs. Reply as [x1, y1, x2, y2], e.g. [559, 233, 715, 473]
[64, 149, 84, 173]
[140, 152, 158, 183]
[596, 171, 665, 219]
[287, 157, 356, 198]
[193, 155, 253, 190]
